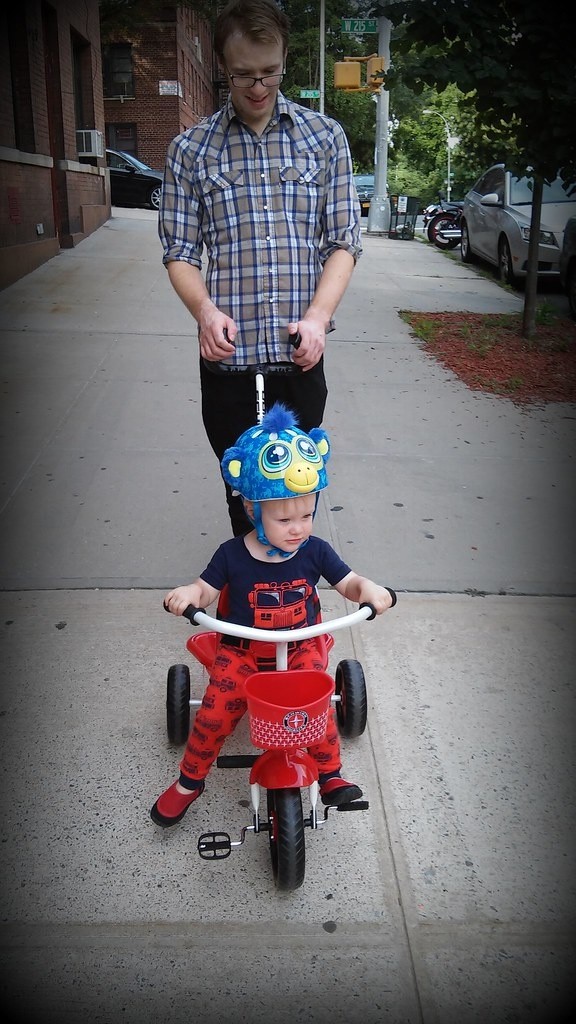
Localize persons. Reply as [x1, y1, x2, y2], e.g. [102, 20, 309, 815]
[151, 405, 392, 828]
[158, 0, 366, 538]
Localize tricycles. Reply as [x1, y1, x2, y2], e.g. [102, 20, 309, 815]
[165, 323, 399, 897]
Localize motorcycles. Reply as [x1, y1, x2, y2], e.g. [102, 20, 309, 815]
[422, 200, 465, 249]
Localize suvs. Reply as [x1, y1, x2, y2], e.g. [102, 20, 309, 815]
[354, 174, 391, 215]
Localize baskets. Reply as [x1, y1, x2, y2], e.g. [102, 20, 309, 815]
[243, 670, 336, 751]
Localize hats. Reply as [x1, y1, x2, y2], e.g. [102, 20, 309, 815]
[220, 405, 329, 500]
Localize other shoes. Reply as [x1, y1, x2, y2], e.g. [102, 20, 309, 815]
[150, 775, 205, 828]
[320, 778, 362, 806]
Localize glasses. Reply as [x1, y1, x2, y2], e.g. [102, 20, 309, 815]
[222, 56, 287, 89]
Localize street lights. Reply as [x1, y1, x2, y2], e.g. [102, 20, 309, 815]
[421, 109, 452, 205]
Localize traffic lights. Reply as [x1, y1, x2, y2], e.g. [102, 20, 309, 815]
[367, 57, 386, 88]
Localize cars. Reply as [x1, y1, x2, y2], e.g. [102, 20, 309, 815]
[557, 216, 576, 324]
[460, 160, 576, 288]
[106, 147, 166, 211]
